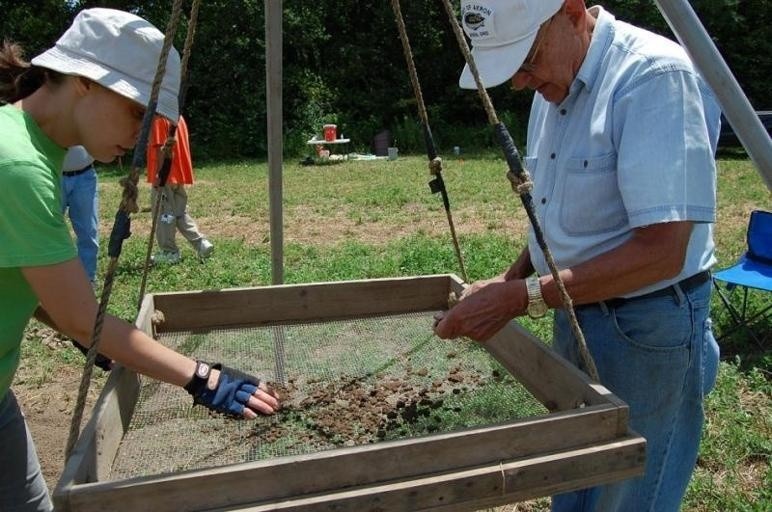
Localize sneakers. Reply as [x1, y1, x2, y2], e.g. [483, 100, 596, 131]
[196, 238, 214, 258]
[144, 250, 181, 264]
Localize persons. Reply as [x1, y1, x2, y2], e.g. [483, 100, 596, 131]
[146, 107, 215, 262]
[431, 0, 721, 512]
[1, 4, 280, 511]
[60, 146, 99, 284]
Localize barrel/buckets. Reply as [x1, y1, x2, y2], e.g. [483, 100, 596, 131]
[454, 146, 460, 154]
[323, 123, 337, 141]
[388, 147, 398, 159]
[375, 134, 390, 155]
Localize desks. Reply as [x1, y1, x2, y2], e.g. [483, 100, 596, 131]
[307, 139, 350, 162]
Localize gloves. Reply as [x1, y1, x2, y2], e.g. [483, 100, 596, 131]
[71, 338, 111, 373]
[182, 355, 262, 421]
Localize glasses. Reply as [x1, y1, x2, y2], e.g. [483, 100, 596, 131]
[521, 14, 555, 72]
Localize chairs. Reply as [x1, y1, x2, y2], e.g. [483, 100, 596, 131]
[712, 206, 771, 356]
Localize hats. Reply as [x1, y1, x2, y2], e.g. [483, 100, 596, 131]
[455, 0, 569, 93]
[27, 6, 186, 128]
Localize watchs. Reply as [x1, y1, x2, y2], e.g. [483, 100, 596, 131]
[524, 276, 547, 319]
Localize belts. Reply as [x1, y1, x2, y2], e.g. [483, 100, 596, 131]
[570, 264, 713, 312]
[60, 163, 94, 176]
